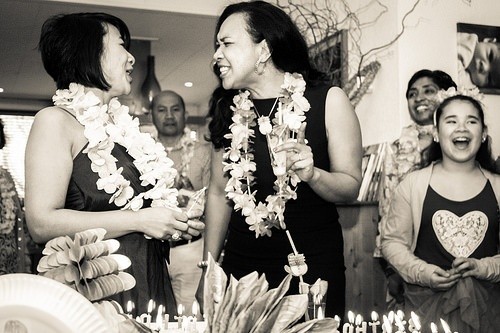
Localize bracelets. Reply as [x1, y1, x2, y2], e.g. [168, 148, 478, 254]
[196, 260, 220, 268]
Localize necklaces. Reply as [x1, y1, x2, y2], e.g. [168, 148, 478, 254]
[52, 71, 311, 240]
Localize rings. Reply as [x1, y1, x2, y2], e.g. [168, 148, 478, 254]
[172, 230, 180, 241]
[296, 152, 301, 162]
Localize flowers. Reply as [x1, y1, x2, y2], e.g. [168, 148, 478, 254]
[436, 86, 485, 103]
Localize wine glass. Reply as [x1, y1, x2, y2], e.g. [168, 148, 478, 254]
[265, 124, 289, 199]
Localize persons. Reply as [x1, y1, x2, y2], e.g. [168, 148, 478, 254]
[149, 89, 211, 319]
[24, 12, 205, 324]
[0, 119, 30, 276]
[195, 0, 363, 333]
[457, 31, 500, 90]
[373, 70, 500, 333]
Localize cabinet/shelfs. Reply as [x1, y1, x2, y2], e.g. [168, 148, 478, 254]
[336, 201, 387, 333]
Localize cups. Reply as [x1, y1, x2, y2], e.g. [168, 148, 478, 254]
[301, 284, 325, 319]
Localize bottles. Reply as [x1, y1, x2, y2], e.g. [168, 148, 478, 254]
[141, 56, 162, 110]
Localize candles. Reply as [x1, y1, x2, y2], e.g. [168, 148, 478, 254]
[126, 299, 200, 333]
[342, 311, 457, 333]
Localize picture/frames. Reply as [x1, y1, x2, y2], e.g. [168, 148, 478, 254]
[308, 28, 349, 90]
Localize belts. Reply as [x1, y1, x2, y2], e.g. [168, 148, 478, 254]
[168, 233, 202, 247]
